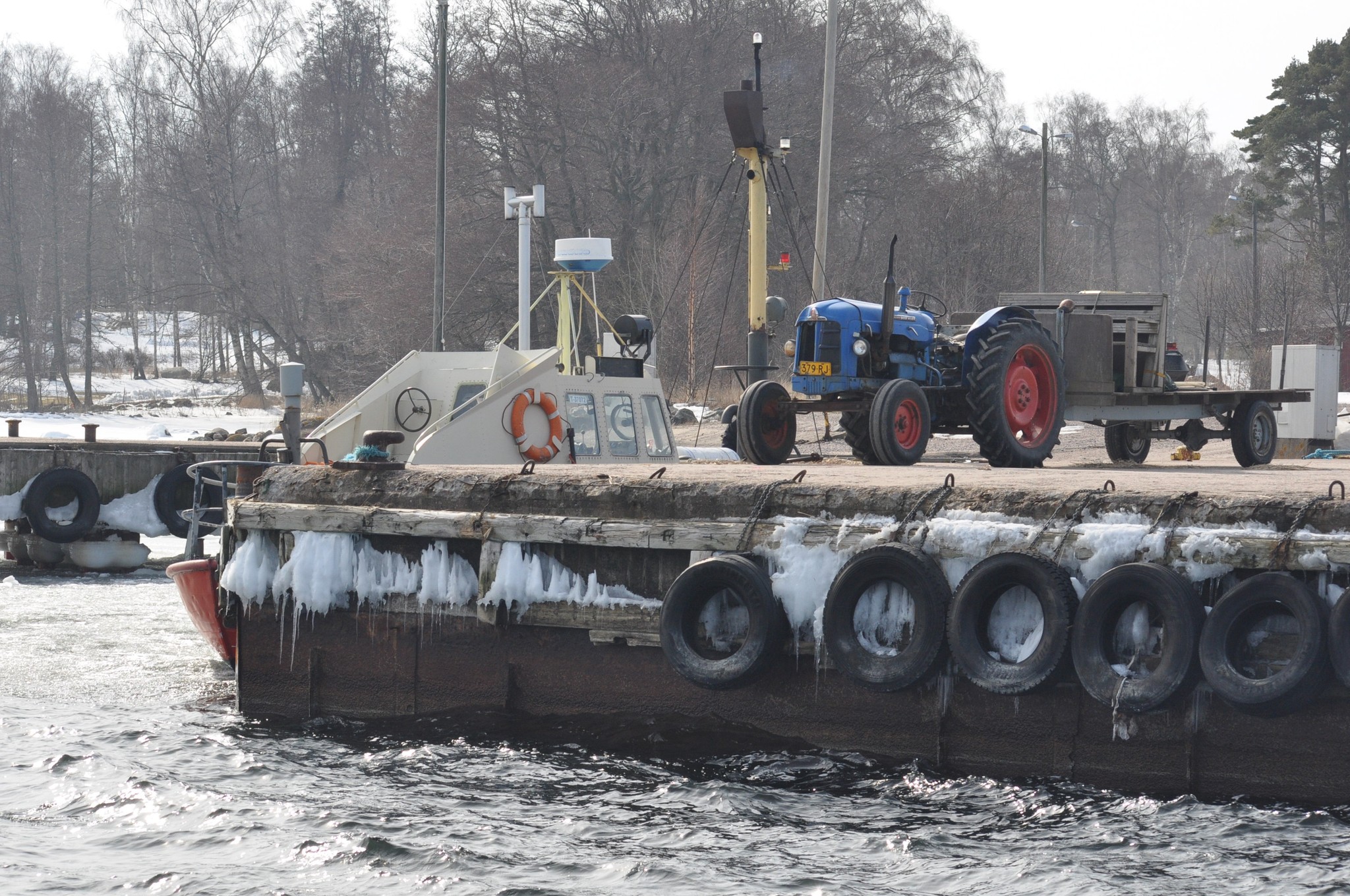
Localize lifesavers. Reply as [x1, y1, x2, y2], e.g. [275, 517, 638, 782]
[1069, 561, 1207, 713]
[24, 465, 102, 544]
[1328, 587, 1350, 687]
[659, 552, 786, 689]
[946, 548, 1081, 696]
[510, 389, 562, 462]
[153, 462, 225, 539]
[822, 541, 953, 692]
[1198, 569, 1328, 714]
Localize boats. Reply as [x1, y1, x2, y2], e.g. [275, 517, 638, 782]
[166, 182, 739, 671]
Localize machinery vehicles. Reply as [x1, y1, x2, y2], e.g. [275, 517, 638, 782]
[732, 233, 1316, 466]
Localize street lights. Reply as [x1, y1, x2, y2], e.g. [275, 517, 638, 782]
[1018, 120, 1074, 293]
[1227, 194, 1259, 342]
